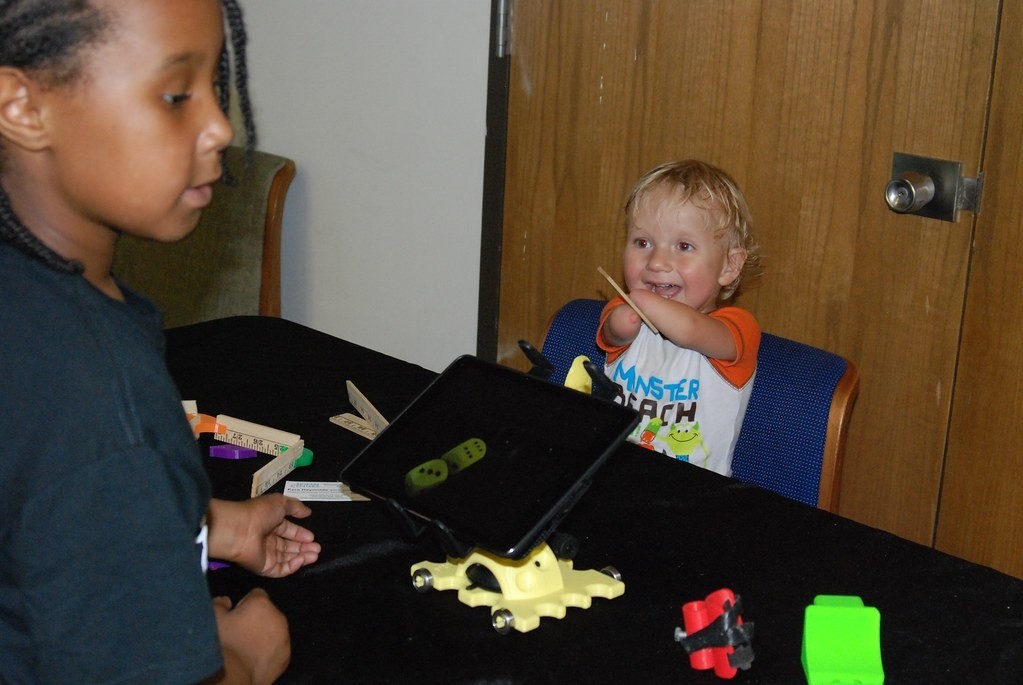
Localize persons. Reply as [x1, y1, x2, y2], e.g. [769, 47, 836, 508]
[0, 2, 320, 685]
[596, 159, 759, 479]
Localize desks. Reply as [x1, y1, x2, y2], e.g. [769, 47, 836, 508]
[163, 314, 1023, 684]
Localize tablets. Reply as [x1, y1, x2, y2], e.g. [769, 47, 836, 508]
[340, 352, 644, 560]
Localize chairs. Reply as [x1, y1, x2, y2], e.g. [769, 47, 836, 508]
[110, 144, 297, 325]
[517, 298, 861, 515]
